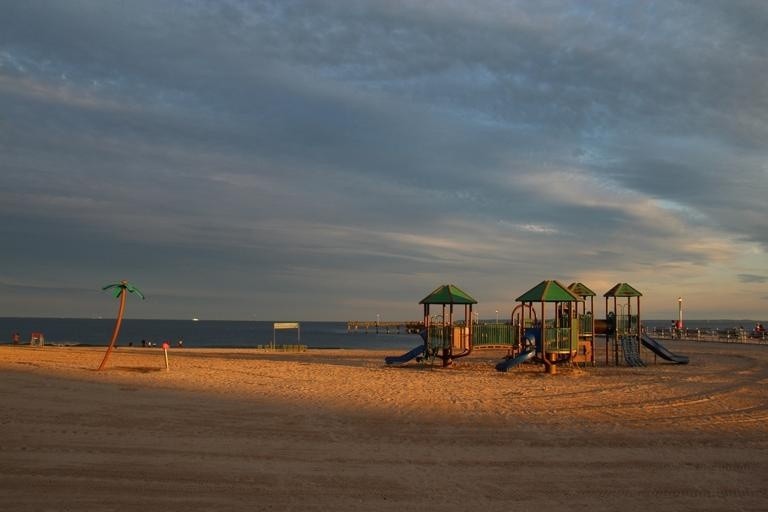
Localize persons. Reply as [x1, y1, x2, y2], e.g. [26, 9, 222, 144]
[740, 323, 768, 343]
[671, 320, 682, 338]
